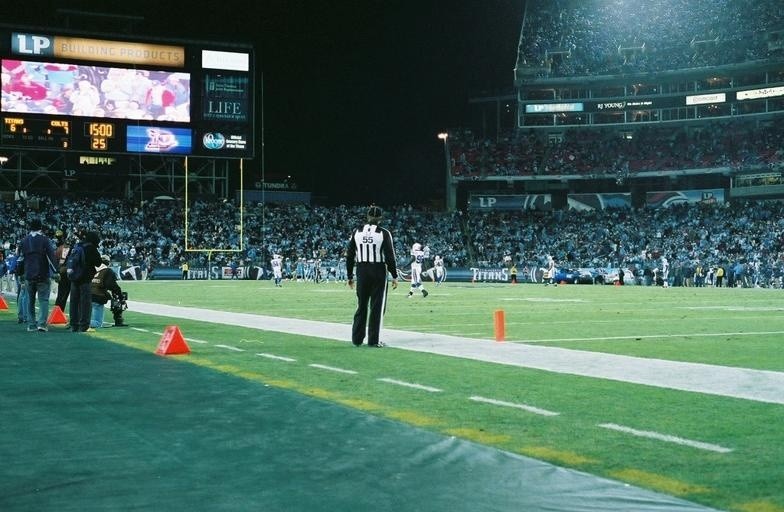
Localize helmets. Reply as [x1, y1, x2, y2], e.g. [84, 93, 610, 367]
[412, 243, 421, 250]
[435, 255, 439, 260]
[275, 255, 278, 259]
[548, 256, 552, 260]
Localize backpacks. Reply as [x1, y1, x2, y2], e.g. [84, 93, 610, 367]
[67, 244, 92, 280]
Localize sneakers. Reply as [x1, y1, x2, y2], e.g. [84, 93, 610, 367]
[422, 290, 427, 297]
[86, 328, 95, 332]
[18, 319, 48, 332]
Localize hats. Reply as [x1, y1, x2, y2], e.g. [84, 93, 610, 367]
[369, 207, 383, 217]
[101, 255, 110, 261]
